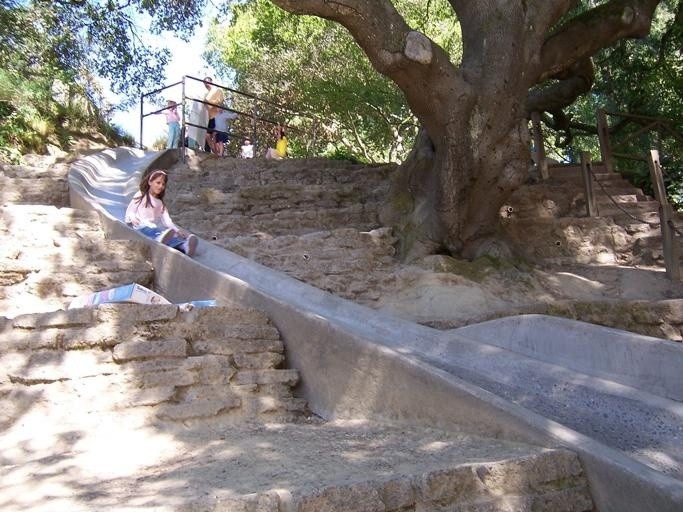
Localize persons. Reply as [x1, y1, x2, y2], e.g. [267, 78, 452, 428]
[266, 131, 288, 160]
[212, 103, 240, 156]
[202, 77, 223, 152]
[152, 100, 181, 150]
[123, 168, 199, 258]
[239, 136, 254, 159]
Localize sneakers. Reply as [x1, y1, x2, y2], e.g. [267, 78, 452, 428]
[184, 234, 198, 257]
[155, 228, 175, 244]
